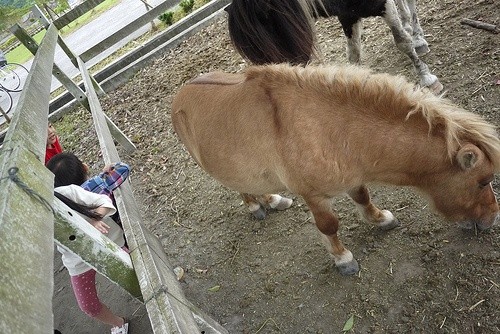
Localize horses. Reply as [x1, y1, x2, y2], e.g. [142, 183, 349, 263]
[170, 64, 499, 276]
[227, 0, 445, 97]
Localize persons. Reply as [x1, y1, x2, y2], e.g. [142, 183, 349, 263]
[45, 120, 131, 334]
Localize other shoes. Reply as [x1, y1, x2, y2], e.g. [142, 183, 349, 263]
[111, 317, 130, 334]
[174, 267, 184, 280]
